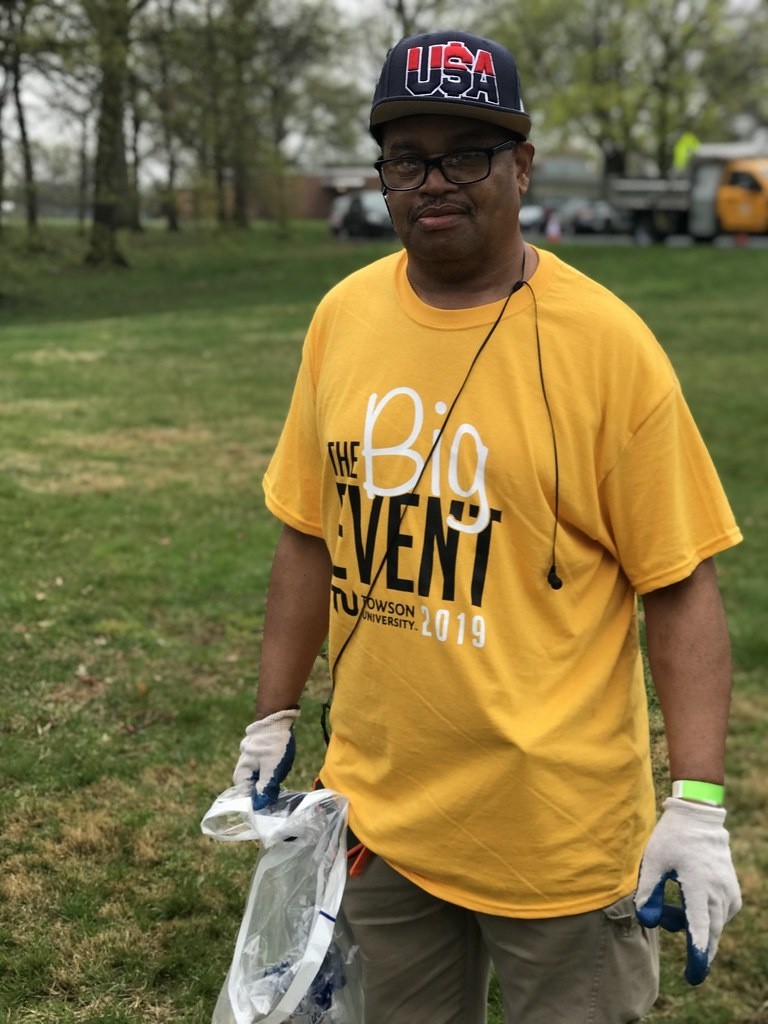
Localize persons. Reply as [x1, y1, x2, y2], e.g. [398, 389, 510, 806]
[232, 33, 742, 1024]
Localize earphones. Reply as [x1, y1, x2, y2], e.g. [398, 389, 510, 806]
[547, 565, 562, 590]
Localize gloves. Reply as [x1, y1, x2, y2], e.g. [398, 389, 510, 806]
[632, 797, 743, 985]
[230, 705, 301, 811]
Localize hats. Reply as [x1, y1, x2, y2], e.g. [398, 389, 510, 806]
[370, 31, 533, 147]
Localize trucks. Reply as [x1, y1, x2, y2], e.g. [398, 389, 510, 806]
[608, 143, 768, 245]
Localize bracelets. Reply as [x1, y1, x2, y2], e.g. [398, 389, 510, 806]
[673, 780, 725, 806]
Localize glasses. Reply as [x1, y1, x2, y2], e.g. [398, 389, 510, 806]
[373, 138, 516, 192]
